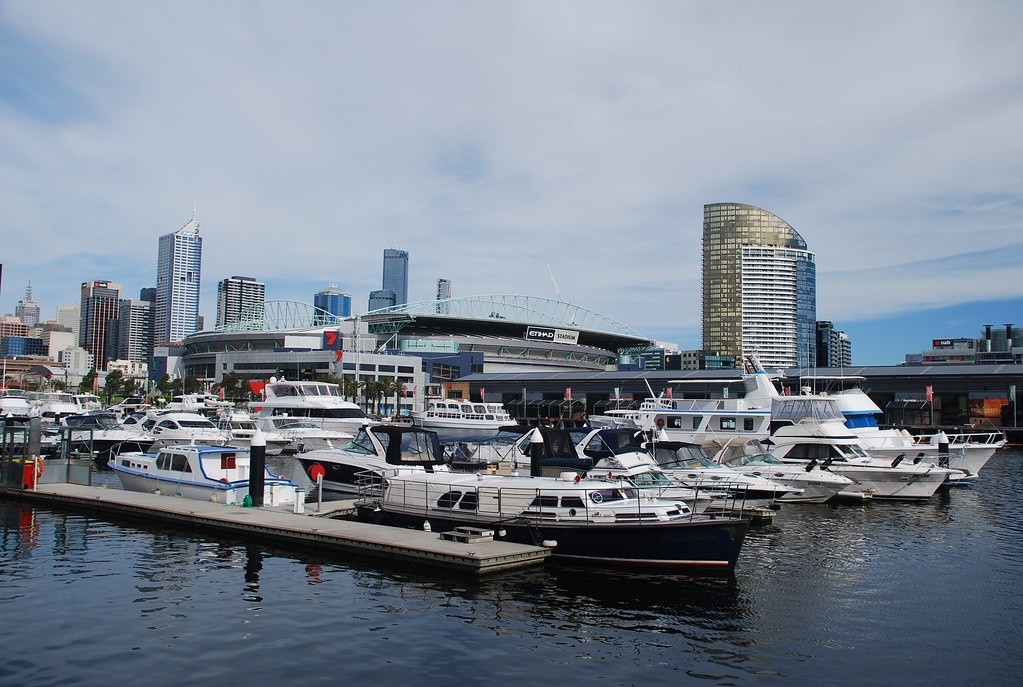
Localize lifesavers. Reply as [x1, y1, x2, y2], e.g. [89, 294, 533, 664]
[31, 456, 45, 479]
[657, 418, 665, 427]
[592, 491, 603, 504]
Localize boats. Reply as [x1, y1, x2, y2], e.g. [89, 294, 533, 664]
[105, 438, 304, 507]
[351, 459, 752, 578]
[1, 363, 1008, 575]
[291, 418, 458, 496]
